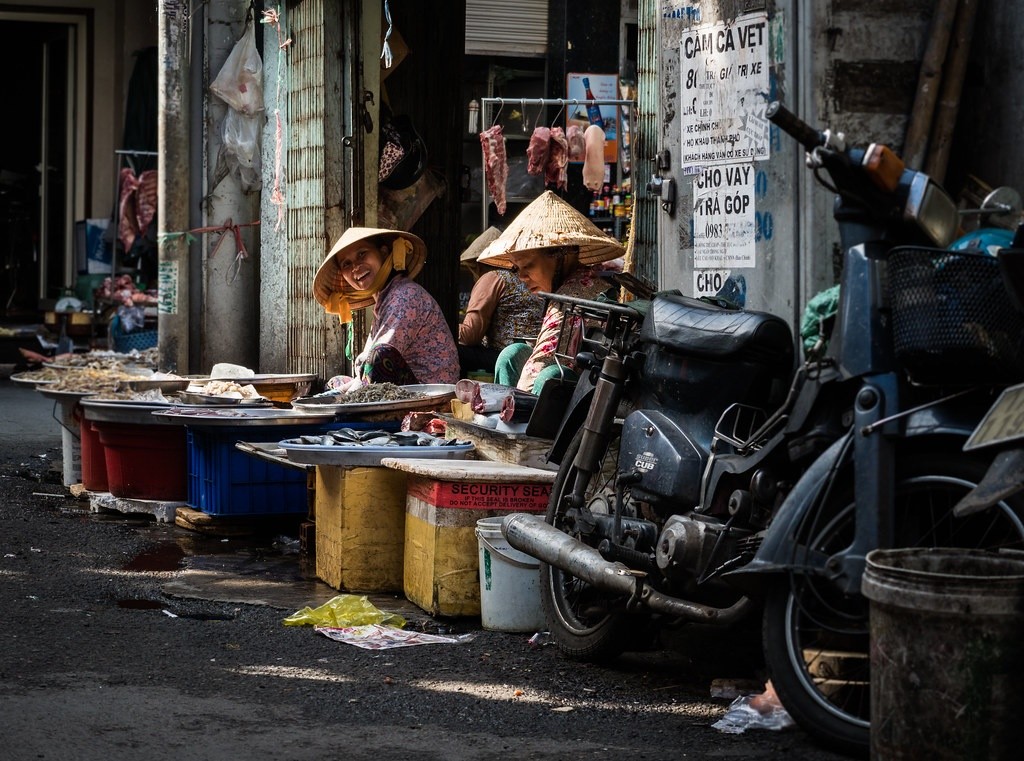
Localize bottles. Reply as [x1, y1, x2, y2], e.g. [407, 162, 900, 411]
[588, 184, 634, 221]
[583, 78, 605, 132]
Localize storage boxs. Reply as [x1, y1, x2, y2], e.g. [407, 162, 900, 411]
[402, 473, 558, 615]
[185, 429, 307, 513]
[313, 468, 411, 591]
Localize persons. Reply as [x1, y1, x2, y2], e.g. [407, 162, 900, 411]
[477, 191, 626, 393]
[458, 226, 546, 379]
[313, 227, 459, 394]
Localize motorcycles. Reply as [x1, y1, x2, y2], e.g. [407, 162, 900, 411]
[500, 97, 1024, 751]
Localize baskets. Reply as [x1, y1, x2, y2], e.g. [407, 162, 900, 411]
[885, 246, 1023, 389]
[114, 330, 157, 354]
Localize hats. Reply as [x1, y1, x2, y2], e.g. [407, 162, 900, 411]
[476, 189, 626, 272]
[313, 227, 426, 312]
[460, 226, 503, 267]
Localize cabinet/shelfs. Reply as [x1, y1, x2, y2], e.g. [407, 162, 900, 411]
[479, 64, 547, 209]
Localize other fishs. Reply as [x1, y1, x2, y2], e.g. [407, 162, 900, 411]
[235, 378, 538, 458]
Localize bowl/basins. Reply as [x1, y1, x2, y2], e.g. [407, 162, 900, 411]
[90, 423, 186, 501]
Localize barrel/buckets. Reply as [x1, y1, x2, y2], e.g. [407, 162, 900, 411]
[475, 514, 557, 634]
[859, 546, 1024, 761]
[53, 399, 81, 487]
[73, 401, 110, 491]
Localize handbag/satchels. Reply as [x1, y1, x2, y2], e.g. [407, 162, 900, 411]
[210, 8, 263, 118]
[360, 344, 420, 386]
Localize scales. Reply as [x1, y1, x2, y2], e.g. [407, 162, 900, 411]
[55, 285, 82, 313]
